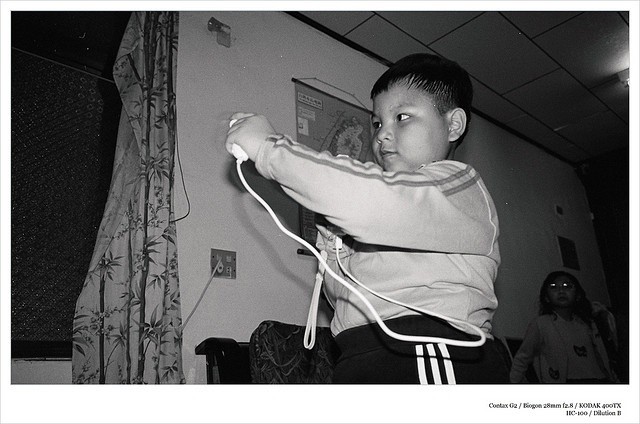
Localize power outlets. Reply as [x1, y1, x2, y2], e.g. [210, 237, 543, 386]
[211, 249, 236, 277]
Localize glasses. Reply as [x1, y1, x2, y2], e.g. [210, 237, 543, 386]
[547, 282, 573, 290]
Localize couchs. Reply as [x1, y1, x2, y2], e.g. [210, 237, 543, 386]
[194, 319, 536, 383]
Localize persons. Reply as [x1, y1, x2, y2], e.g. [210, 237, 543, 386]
[223, 52, 501, 383]
[511, 269, 623, 383]
